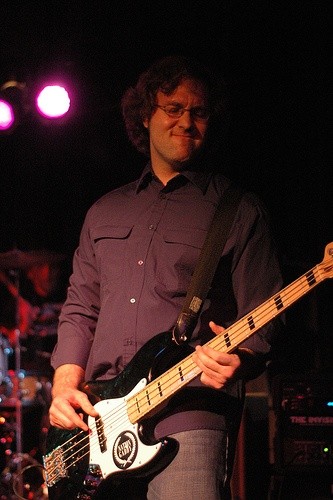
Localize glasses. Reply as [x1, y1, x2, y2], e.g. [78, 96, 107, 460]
[151, 104, 211, 122]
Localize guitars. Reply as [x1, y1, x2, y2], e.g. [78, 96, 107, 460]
[51, 243, 333, 500]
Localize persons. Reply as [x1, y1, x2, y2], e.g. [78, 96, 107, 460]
[45, 53, 293, 500]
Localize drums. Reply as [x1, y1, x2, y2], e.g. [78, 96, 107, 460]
[0, 371, 48, 407]
